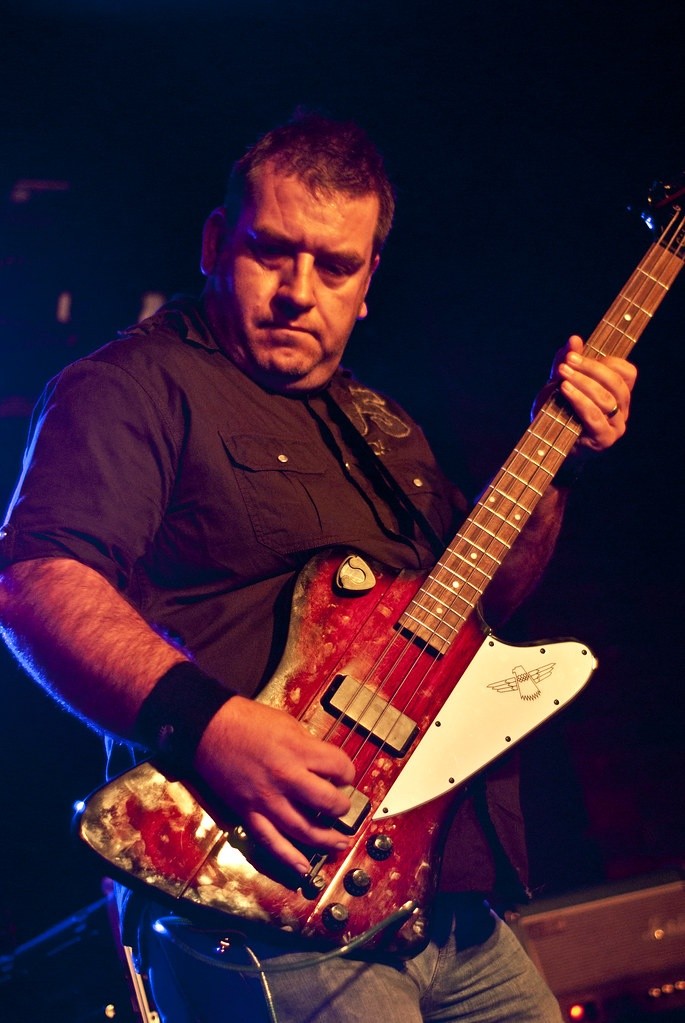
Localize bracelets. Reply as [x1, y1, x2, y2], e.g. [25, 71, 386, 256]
[132, 661, 237, 773]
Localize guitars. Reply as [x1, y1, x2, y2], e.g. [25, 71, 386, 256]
[62, 190, 685, 964]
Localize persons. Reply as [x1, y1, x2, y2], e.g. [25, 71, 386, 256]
[0, 115, 638, 1023]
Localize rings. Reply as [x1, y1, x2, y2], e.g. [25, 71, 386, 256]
[605, 404, 617, 419]
